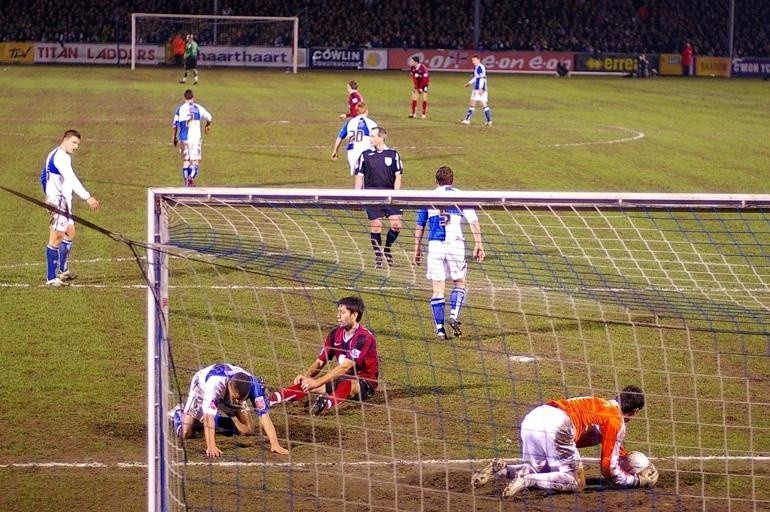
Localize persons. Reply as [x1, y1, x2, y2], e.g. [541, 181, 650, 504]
[409, 56, 430, 119]
[355, 127, 403, 269]
[478, 0, 770, 56]
[178, 35, 198, 85]
[259, 297, 378, 416]
[173, 90, 212, 187]
[339, 81, 363, 122]
[472, 384, 658, 502]
[297, 0, 477, 50]
[556, 58, 568, 77]
[41, 129, 99, 288]
[459, 55, 492, 127]
[168, 363, 290, 458]
[639, 53, 649, 78]
[331, 103, 378, 189]
[0, 0, 297, 45]
[413, 165, 485, 341]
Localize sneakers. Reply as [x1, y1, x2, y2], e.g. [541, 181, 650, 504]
[178, 80, 186, 85]
[484, 121, 491, 127]
[420, 114, 426, 119]
[460, 120, 469, 125]
[257, 375, 267, 392]
[46, 278, 70, 290]
[408, 113, 416, 119]
[501, 463, 532, 502]
[384, 251, 394, 266]
[446, 317, 463, 338]
[438, 334, 450, 342]
[310, 391, 329, 417]
[193, 81, 199, 85]
[376, 253, 384, 268]
[167, 401, 185, 421]
[57, 270, 78, 282]
[470, 457, 507, 486]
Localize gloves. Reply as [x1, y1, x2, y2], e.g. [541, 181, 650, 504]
[632, 464, 658, 491]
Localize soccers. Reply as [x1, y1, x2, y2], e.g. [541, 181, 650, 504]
[621, 450, 650, 476]
